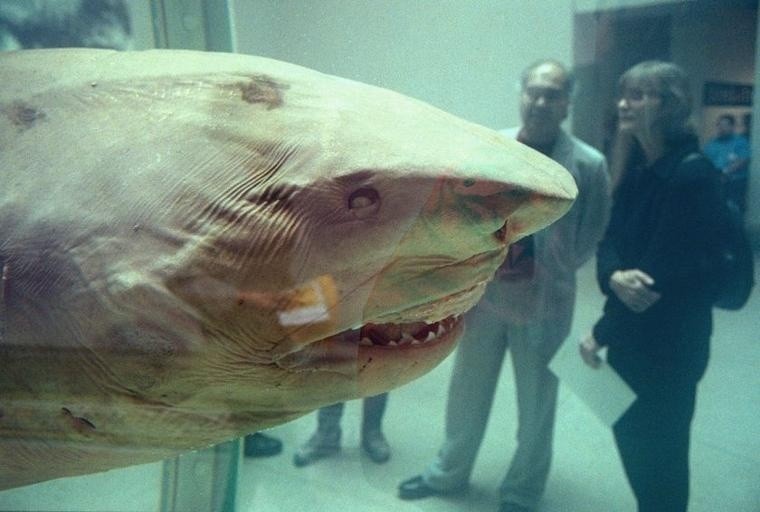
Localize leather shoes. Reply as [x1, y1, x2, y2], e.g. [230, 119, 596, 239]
[365, 433, 391, 463]
[293, 439, 340, 466]
[399, 475, 434, 498]
[497, 502, 529, 512]
[245, 432, 282, 457]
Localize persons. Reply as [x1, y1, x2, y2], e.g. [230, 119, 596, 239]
[244, 392, 390, 467]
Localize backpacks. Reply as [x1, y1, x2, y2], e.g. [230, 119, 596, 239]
[712, 166, 754, 311]
